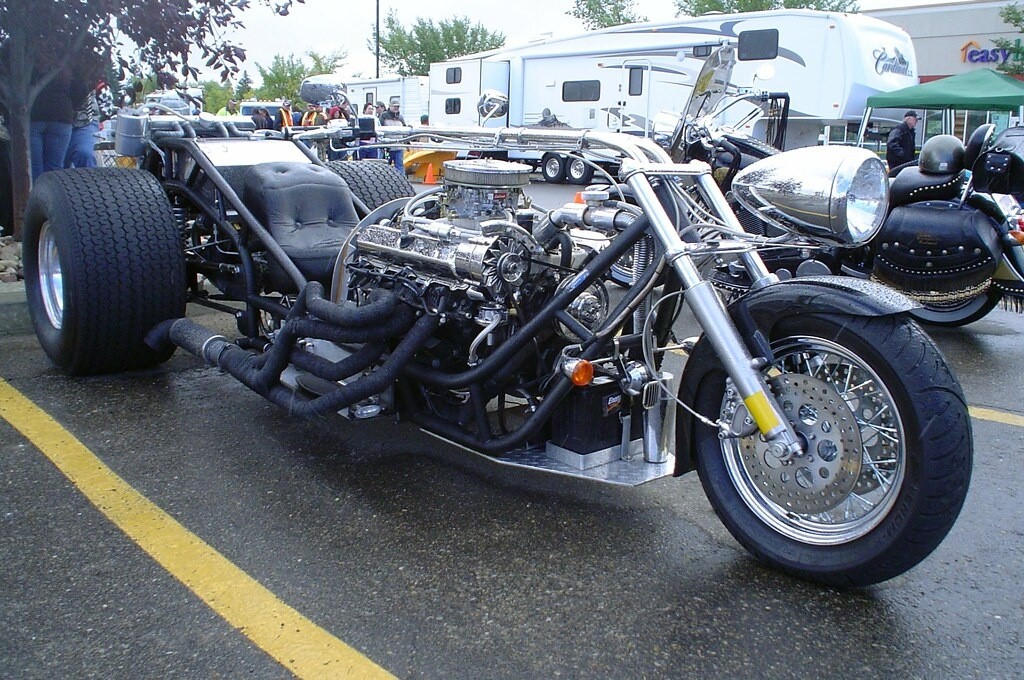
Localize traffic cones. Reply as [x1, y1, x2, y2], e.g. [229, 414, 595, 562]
[421, 163, 436, 186]
[572, 191, 585, 204]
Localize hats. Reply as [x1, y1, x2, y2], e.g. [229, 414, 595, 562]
[229, 98, 237, 104]
[905, 110, 922, 120]
[390, 99, 400, 107]
[283, 99, 291, 106]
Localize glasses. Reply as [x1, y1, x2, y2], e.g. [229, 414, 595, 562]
[394, 105, 399, 107]
[306, 105, 316, 107]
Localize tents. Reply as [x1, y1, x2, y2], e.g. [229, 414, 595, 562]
[857, 68, 1024, 175]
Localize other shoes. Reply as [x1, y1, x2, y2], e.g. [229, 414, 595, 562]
[0, 228, 13, 237]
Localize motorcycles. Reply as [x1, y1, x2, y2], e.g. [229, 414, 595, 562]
[18, 30, 1024, 593]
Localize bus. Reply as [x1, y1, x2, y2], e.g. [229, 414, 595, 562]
[428, 10, 921, 186]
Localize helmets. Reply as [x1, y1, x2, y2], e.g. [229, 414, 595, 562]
[918, 135, 965, 176]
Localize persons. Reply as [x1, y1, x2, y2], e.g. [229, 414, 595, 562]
[214, 98, 429, 177]
[886, 110, 922, 178]
[537, 107, 551, 126]
[0, 61, 114, 237]
